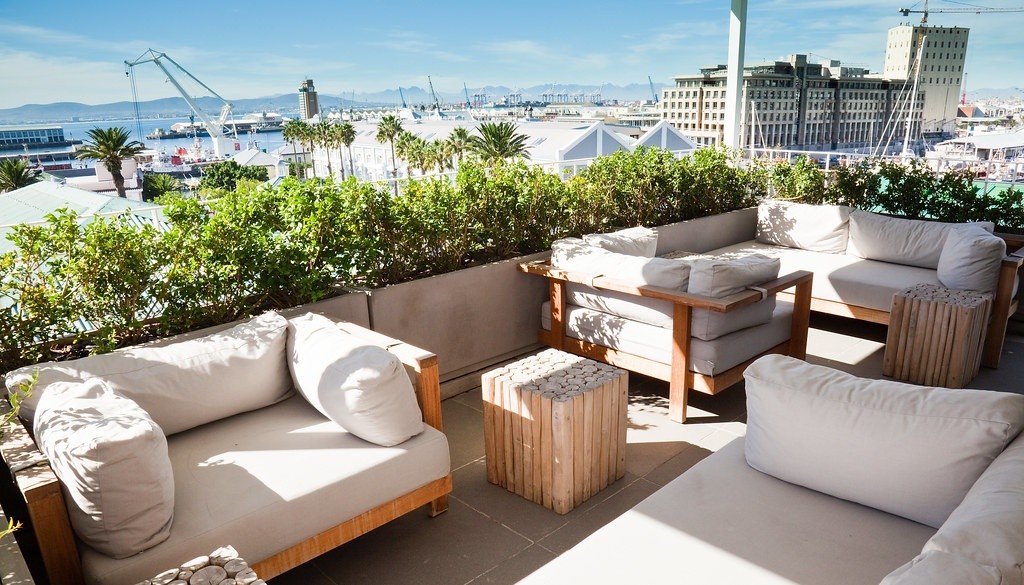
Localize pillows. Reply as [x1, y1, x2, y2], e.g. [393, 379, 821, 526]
[286, 311, 424, 447]
[755, 198, 855, 254]
[846, 208, 995, 269]
[920, 429, 1024, 585]
[32, 378, 176, 560]
[3, 309, 296, 435]
[879, 549, 1002, 585]
[742, 353, 1024, 533]
[585, 225, 658, 256]
[687, 252, 780, 340]
[937, 225, 1007, 293]
[550, 235, 691, 328]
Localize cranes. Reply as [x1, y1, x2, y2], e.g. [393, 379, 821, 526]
[898, 0, 1024, 78]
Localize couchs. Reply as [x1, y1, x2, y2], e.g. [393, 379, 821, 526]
[516, 224, 813, 423]
[699, 198, 1024, 370]
[508, 353, 1024, 585]
[0, 308, 453, 585]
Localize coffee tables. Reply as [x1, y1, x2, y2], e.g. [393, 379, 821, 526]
[659, 248, 695, 259]
[133, 544, 268, 585]
[481, 347, 630, 515]
[882, 282, 993, 389]
